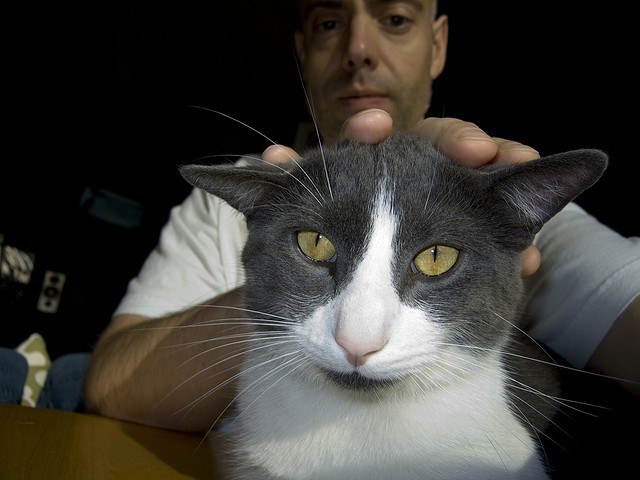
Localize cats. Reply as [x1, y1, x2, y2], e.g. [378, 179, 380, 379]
[133, 91, 631, 479]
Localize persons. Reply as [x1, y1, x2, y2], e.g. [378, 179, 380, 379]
[82, 0, 639, 435]
